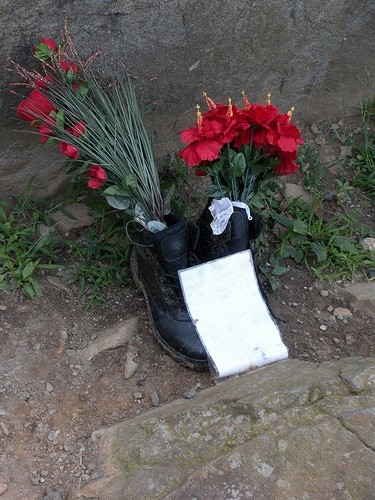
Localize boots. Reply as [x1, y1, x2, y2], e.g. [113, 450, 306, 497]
[126, 210, 215, 370]
[189, 197, 275, 321]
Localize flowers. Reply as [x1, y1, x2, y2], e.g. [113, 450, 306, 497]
[175, 91, 307, 238]
[7, 15, 178, 248]
